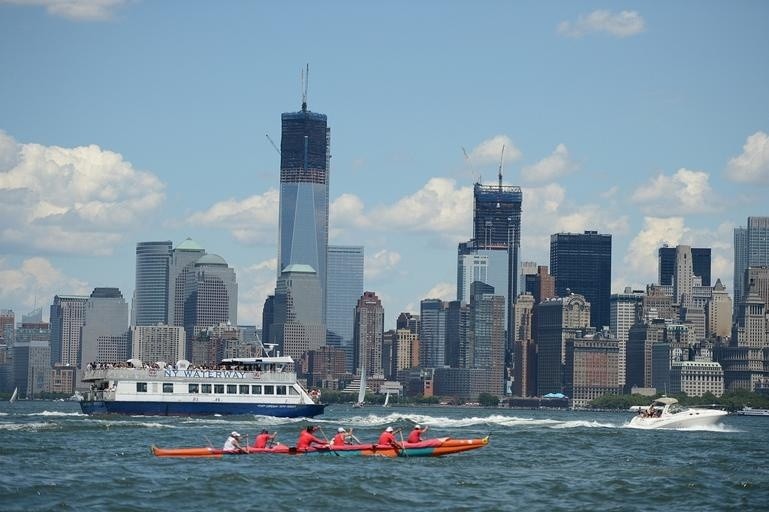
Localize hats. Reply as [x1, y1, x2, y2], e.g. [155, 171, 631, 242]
[231, 432, 241, 437]
[262, 428, 269, 434]
[385, 427, 393, 432]
[306, 425, 313, 430]
[414, 425, 420, 428]
[337, 427, 346, 432]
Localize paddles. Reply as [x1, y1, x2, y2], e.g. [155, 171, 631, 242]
[398, 430, 410, 458]
[319, 428, 344, 457]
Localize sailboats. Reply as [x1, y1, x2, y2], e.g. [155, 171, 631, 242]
[354, 365, 391, 408]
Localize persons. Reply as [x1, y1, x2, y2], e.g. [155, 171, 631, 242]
[223, 432, 249, 454]
[638, 405, 662, 418]
[296, 426, 329, 448]
[86, 362, 259, 370]
[330, 427, 352, 445]
[307, 388, 321, 396]
[91, 383, 116, 390]
[408, 425, 428, 443]
[378, 427, 405, 449]
[254, 429, 276, 448]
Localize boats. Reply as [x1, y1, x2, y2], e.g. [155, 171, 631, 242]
[151, 433, 490, 458]
[738, 406, 769, 416]
[79, 342, 324, 417]
[629, 397, 729, 429]
